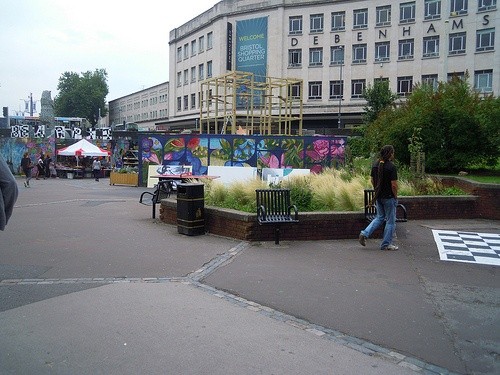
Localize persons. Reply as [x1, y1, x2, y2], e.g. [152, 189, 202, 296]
[56, 161, 66, 177]
[35, 159, 46, 180]
[359, 144, 400, 251]
[21, 152, 33, 188]
[48, 157, 60, 180]
[73, 155, 122, 173]
[93, 157, 101, 182]
[0, 149, 18, 231]
[42, 154, 52, 177]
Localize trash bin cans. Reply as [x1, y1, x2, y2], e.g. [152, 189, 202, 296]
[177, 184, 205, 235]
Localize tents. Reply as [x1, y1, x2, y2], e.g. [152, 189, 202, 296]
[56, 138, 111, 176]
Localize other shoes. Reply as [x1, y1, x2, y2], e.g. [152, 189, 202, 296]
[44, 177, 45, 179]
[26, 185, 30, 188]
[381, 244, 399, 250]
[24, 181, 27, 187]
[36, 178, 40, 180]
[359, 234, 366, 246]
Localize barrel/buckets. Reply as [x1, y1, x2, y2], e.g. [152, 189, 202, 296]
[67, 172, 73, 179]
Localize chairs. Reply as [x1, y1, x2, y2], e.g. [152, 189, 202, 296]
[363, 189, 408, 239]
[256, 188, 300, 245]
[139, 180, 173, 219]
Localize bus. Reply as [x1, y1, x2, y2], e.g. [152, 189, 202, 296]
[10, 116, 86, 129]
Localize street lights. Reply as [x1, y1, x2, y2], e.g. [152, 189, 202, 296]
[338, 45, 344, 129]
[98, 108, 101, 128]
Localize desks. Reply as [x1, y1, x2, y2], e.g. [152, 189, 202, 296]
[102, 169, 112, 178]
[57, 169, 85, 178]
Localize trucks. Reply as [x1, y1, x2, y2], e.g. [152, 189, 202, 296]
[116, 120, 138, 131]
